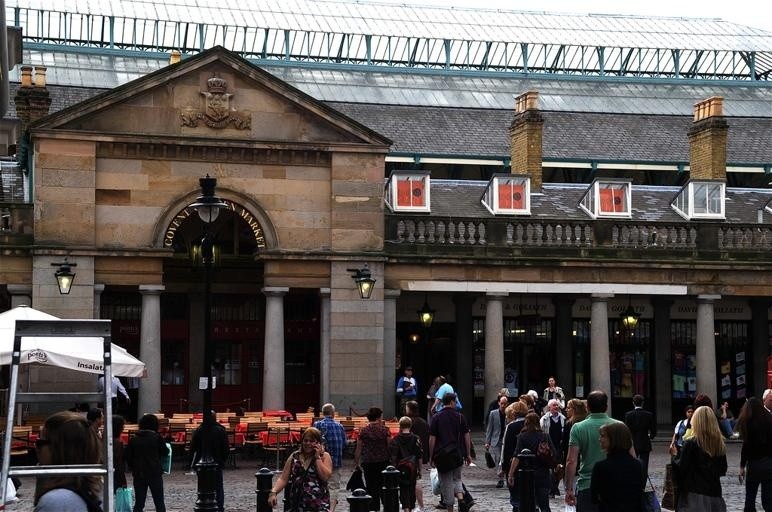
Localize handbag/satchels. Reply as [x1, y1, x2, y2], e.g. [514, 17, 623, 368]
[644, 489, 661, 512]
[346, 469, 366, 491]
[484, 451, 496, 468]
[538, 441, 555, 464]
[662, 485, 678, 510]
[556, 398, 566, 409]
[666, 462, 681, 485]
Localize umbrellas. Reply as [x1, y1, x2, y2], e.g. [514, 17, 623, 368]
[0, 304, 148, 423]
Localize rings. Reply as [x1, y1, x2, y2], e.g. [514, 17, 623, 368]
[320, 447, 321, 449]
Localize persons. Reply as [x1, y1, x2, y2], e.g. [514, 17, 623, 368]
[717, 401, 739, 440]
[431, 376, 462, 415]
[86, 408, 103, 436]
[405, 401, 428, 509]
[99, 376, 131, 414]
[314, 403, 347, 509]
[269, 426, 332, 510]
[126, 414, 168, 509]
[101, 416, 127, 494]
[396, 367, 417, 416]
[670, 393, 728, 509]
[734, 389, 770, 509]
[428, 394, 472, 509]
[34, 411, 101, 510]
[189, 410, 229, 508]
[390, 416, 424, 509]
[670, 405, 694, 454]
[353, 407, 391, 509]
[484, 376, 659, 509]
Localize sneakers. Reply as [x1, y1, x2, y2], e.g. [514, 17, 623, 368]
[404, 491, 474, 512]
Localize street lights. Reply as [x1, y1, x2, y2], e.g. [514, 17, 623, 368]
[190, 171, 228, 511]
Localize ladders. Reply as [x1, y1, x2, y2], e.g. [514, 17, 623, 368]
[0, 319, 115, 512]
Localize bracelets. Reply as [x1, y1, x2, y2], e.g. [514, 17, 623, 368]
[566, 487, 573, 491]
[315, 456, 320, 459]
[272, 491, 277, 493]
[508, 476, 514, 477]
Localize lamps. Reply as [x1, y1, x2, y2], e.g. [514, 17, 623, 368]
[346, 261, 377, 300]
[618, 294, 641, 332]
[51, 256, 79, 296]
[572, 328, 578, 337]
[714, 329, 721, 338]
[414, 292, 437, 328]
